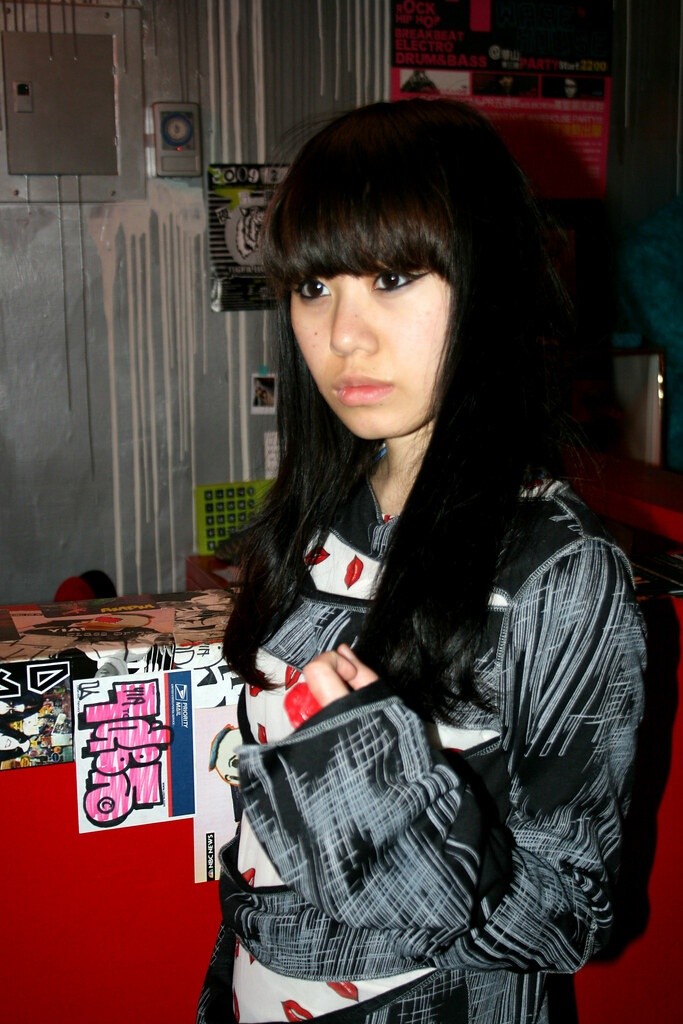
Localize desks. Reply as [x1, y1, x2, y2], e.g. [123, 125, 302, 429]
[0, 559, 683, 1024]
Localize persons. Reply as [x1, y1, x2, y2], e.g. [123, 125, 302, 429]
[196, 95, 650, 1024]
